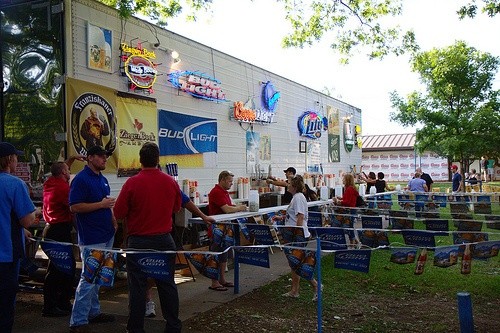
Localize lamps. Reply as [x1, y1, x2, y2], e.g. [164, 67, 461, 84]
[346, 112, 353, 119]
[165, 49, 182, 64]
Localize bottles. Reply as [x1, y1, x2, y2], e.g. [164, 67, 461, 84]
[248, 190, 260, 212]
[320, 186, 328, 201]
[334, 185, 342, 198]
[359, 184, 365, 196]
[95, 254, 116, 285]
[81, 250, 105, 282]
[370, 186, 376, 194]
[396, 185, 401, 191]
[191, 195, 500, 279]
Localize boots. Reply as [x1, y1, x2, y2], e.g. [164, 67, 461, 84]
[41, 277, 72, 317]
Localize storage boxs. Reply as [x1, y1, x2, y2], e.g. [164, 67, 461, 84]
[176, 245, 208, 276]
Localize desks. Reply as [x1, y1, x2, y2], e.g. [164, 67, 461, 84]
[188, 198, 340, 246]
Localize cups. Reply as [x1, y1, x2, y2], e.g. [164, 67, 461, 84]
[303, 173, 335, 188]
[107, 196, 116, 206]
[237, 177, 270, 199]
[183, 179, 199, 205]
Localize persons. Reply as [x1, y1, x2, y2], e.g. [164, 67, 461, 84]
[209, 171, 248, 291]
[333, 173, 364, 250]
[68, 145, 119, 333]
[358, 171, 395, 195]
[451, 164, 462, 192]
[115, 143, 187, 333]
[80, 107, 109, 148]
[405, 168, 434, 220]
[268, 167, 296, 206]
[156, 162, 215, 251]
[292, 173, 318, 203]
[465, 156, 495, 185]
[42, 161, 77, 317]
[0, 141, 43, 333]
[272, 175, 323, 300]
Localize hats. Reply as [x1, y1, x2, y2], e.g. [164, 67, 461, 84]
[87, 146, 112, 157]
[283, 167, 296, 174]
[0, 141, 25, 156]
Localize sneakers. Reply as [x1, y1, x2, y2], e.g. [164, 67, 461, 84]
[145, 303, 157, 318]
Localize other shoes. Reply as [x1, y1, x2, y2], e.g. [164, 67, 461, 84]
[70, 326, 96, 333]
[88, 313, 115, 323]
[311, 284, 324, 301]
[282, 291, 300, 298]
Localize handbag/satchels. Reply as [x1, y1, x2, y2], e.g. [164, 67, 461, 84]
[355, 195, 364, 206]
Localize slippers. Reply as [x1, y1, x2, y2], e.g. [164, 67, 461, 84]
[208, 282, 233, 291]
